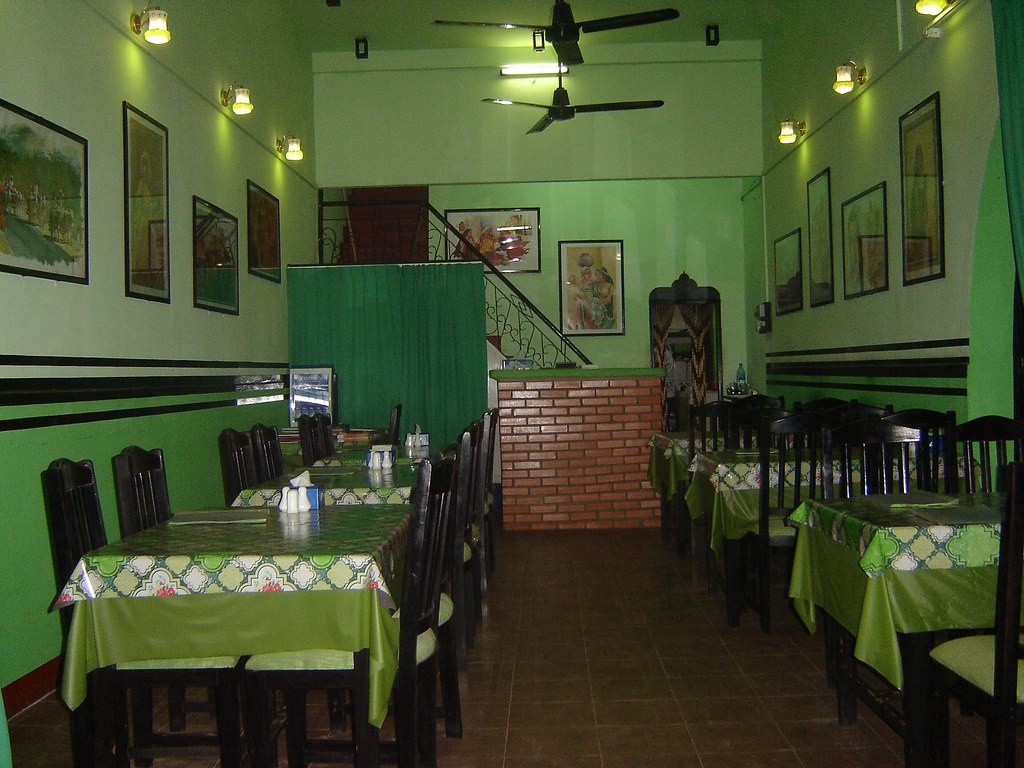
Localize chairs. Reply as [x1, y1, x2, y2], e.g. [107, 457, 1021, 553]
[41, 402, 501, 768]
[665, 395, 1024, 768]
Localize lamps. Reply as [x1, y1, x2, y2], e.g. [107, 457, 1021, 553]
[499, 66, 569, 74]
[221, 82, 253, 114]
[778, 118, 806, 144]
[833, 60, 866, 95]
[915, 0, 955, 16]
[131, 0, 171, 45]
[276, 134, 303, 161]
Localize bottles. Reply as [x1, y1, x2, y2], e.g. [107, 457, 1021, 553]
[382, 451, 392, 469]
[404, 432, 421, 448]
[725, 363, 752, 396]
[278, 486, 290, 513]
[298, 487, 311, 513]
[287, 489, 299, 513]
[368, 451, 382, 469]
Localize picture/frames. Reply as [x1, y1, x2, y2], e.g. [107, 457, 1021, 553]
[444, 207, 542, 273]
[246, 178, 281, 284]
[193, 194, 239, 316]
[773, 228, 804, 316]
[148, 220, 168, 270]
[806, 167, 835, 309]
[898, 91, 946, 288]
[905, 236, 933, 282]
[840, 180, 889, 300]
[0, 98, 90, 285]
[122, 99, 171, 305]
[557, 240, 625, 336]
[857, 235, 887, 291]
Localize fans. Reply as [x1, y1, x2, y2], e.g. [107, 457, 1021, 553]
[480, 57, 664, 134]
[433, 0, 680, 66]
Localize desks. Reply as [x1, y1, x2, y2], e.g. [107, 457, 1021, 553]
[231, 464, 420, 505]
[646, 430, 810, 563]
[787, 491, 1024, 766]
[313, 443, 451, 466]
[684, 445, 983, 629]
[50, 504, 419, 768]
[278, 432, 390, 473]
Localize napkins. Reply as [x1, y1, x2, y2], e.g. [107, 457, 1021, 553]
[287, 468, 361, 474]
[168, 508, 270, 526]
[877, 490, 960, 508]
[735, 446, 779, 455]
